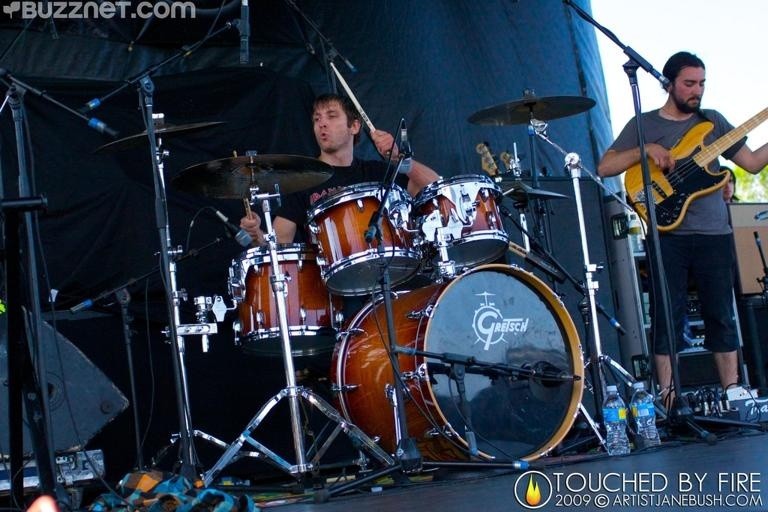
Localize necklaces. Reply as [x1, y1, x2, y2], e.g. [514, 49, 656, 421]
[661, 107, 693, 121]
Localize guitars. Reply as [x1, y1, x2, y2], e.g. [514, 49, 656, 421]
[625, 107, 768, 230]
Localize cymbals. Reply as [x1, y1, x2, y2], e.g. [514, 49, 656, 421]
[101, 118, 226, 152]
[467, 95, 598, 126]
[507, 185, 573, 200]
[179, 152, 334, 199]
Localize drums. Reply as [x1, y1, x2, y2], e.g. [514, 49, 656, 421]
[406, 172, 508, 265]
[331, 266, 587, 461]
[306, 180, 423, 297]
[232, 243, 341, 358]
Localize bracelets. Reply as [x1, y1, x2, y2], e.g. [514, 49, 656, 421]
[396, 149, 411, 173]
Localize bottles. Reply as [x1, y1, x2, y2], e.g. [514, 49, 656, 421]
[602, 386, 631, 455]
[629, 214, 644, 253]
[630, 382, 661, 446]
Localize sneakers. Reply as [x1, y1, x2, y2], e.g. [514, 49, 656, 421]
[375, 475, 394, 486]
[409, 474, 433, 483]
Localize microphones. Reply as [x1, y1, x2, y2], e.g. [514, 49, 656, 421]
[50, 16, 57, 41]
[399, 117, 411, 174]
[239, 1, 249, 64]
[206, 205, 253, 249]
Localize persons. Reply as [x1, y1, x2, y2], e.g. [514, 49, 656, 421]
[597, 51, 768, 437]
[238, 94, 444, 487]
[720, 166, 737, 202]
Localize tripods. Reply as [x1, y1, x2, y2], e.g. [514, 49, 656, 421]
[314, 238, 529, 502]
[621, 62, 767, 446]
[139, 90, 300, 494]
[552, 153, 671, 457]
[194, 200, 412, 490]
